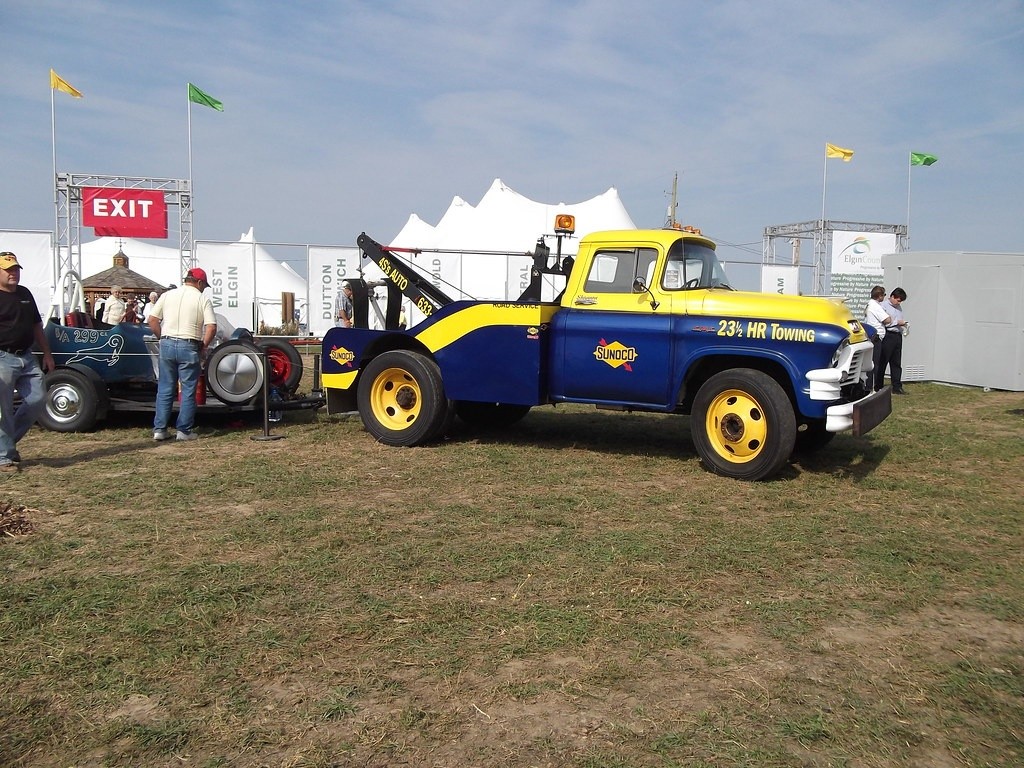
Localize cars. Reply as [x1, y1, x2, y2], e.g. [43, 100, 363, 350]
[31, 273, 301, 436]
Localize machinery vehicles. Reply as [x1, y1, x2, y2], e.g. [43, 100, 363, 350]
[316, 216, 896, 481]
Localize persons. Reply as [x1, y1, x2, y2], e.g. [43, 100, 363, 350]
[864, 286, 891, 393]
[147, 268, 217, 441]
[398, 307, 407, 330]
[95, 285, 158, 326]
[0, 252, 55, 467]
[879, 287, 910, 396]
[335, 285, 353, 328]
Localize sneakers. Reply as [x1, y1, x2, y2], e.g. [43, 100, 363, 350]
[175, 426, 199, 440]
[154, 428, 172, 441]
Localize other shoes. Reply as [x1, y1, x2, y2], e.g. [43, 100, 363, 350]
[892, 388, 910, 396]
[13, 450, 21, 462]
[0, 465, 19, 475]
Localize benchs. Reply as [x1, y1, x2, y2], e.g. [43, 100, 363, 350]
[64, 312, 94, 329]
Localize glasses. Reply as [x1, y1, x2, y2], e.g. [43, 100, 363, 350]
[0, 252, 15, 256]
[349, 288, 352, 291]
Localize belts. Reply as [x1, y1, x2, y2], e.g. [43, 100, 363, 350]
[161, 336, 196, 342]
[334, 316, 343, 318]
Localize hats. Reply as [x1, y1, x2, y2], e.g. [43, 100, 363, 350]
[186, 268, 211, 288]
[0, 255, 23, 270]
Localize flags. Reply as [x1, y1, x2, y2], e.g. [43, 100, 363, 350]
[912, 153, 938, 166]
[827, 142, 854, 162]
[189, 81, 223, 112]
[50, 70, 83, 99]
